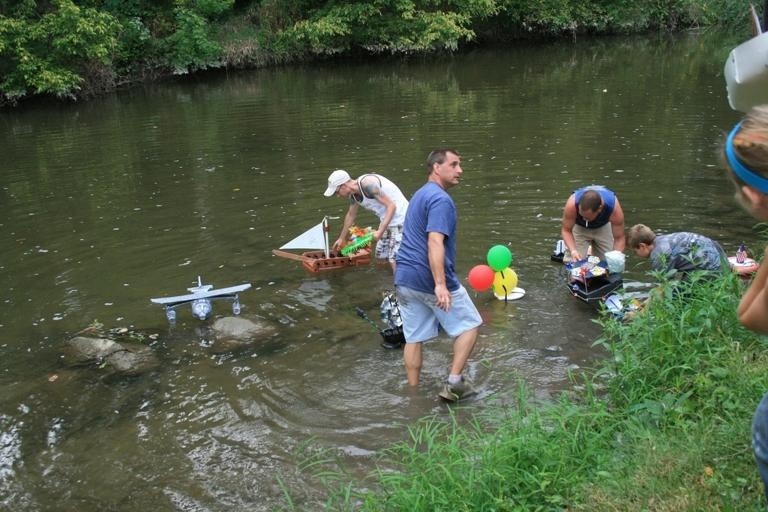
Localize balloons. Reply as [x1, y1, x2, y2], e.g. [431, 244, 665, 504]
[490, 268, 519, 295]
[468, 264, 496, 293]
[486, 245, 513, 275]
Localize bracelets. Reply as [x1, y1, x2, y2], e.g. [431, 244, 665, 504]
[570, 250, 577, 257]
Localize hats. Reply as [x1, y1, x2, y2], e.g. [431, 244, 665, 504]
[323, 170, 352, 197]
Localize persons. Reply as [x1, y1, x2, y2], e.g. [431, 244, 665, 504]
[622, 222, 731, 325]
[391, 147, 485, 404]
[560, 184, 627, 283]
[715, 102, 768, 500]
[322, 169, 410, 278]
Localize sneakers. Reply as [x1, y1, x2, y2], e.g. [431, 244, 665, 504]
[438, 379, 476, 402]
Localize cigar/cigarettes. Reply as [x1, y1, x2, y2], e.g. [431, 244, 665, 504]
[585, 220, 588, 227]
